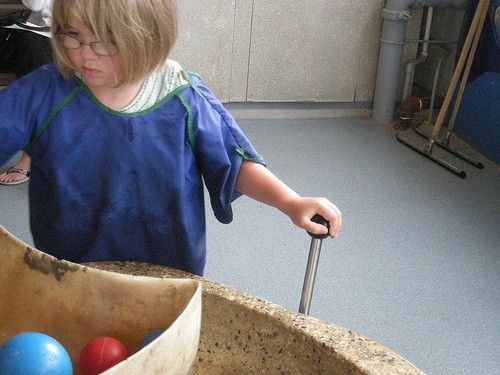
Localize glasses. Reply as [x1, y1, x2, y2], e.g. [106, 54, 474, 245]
[54, 25, 120, 56]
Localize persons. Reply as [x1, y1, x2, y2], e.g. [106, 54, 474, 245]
[0, 0, 343, 278]
[0, 0, 53, 185]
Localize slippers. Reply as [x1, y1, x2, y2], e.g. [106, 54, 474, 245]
[0, 163, 31, 185]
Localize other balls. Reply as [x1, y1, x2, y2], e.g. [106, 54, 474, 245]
[80, 336, 129, 375]
[0, 331, 73, 375]
